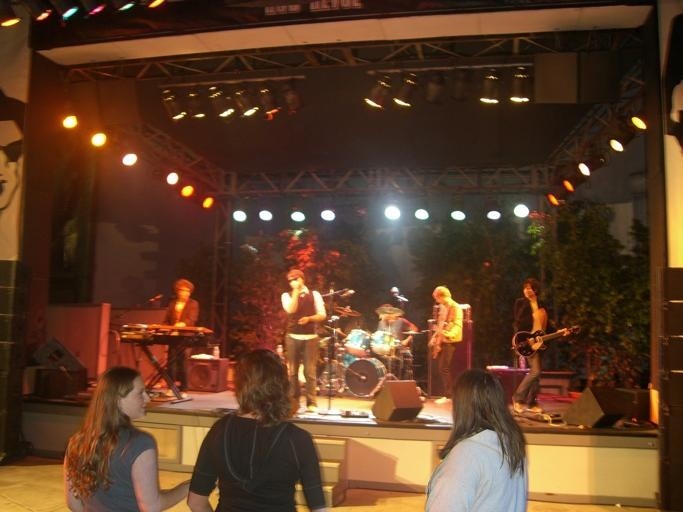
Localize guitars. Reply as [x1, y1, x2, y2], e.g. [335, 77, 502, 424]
[512, 326, 582, 359]
[430, 322, 448, 360]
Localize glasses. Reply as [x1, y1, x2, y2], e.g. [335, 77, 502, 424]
[286, 269, 305, 280]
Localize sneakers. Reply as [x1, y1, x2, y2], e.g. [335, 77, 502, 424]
[163, 388, 190, 399]
[434, 396, 451, 403]
[526, 405, 544, 413]
[511, 395, 524, 413]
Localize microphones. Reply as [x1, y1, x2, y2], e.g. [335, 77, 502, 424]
[341, 289, 356, 298]
[146, 294, 163, 303]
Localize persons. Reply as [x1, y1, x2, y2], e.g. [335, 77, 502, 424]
[161, 278, 199, 398]
[511, 276, 571, 414]
[429, 284, 463, 404]
[186, 348, 329, 512]
[375, 303, 419, 347]
[63, 366, 191, 512]
[424, 367, 529, 512]
[280, 268, 326, 412]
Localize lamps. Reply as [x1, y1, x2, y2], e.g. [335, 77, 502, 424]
[377, 184, 534, 226]
[225, 190, 341, 227]
[359, 54, 535, 116]
[543, 99, 649, 212]
[56, 94, 219, 215]
[149, 66, 308, 129]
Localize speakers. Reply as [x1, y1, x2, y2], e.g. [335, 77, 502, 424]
[371, 379, 424, 422]
[489, 368, 526, 405]
[184, 357, 228, 393]
[564, 387, 629, 429]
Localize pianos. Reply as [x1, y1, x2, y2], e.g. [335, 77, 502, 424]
[120, 323, 216, 347]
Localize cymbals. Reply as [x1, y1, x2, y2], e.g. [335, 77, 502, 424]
[374, 306, 405, 318]
[323, 324, 347, 337]
[334, 306, 361, 317]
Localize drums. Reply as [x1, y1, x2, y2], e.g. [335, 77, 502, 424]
[344, 357, 388, 398]
[371, 331, 395, 355]
[343, 329, 372, 356]
